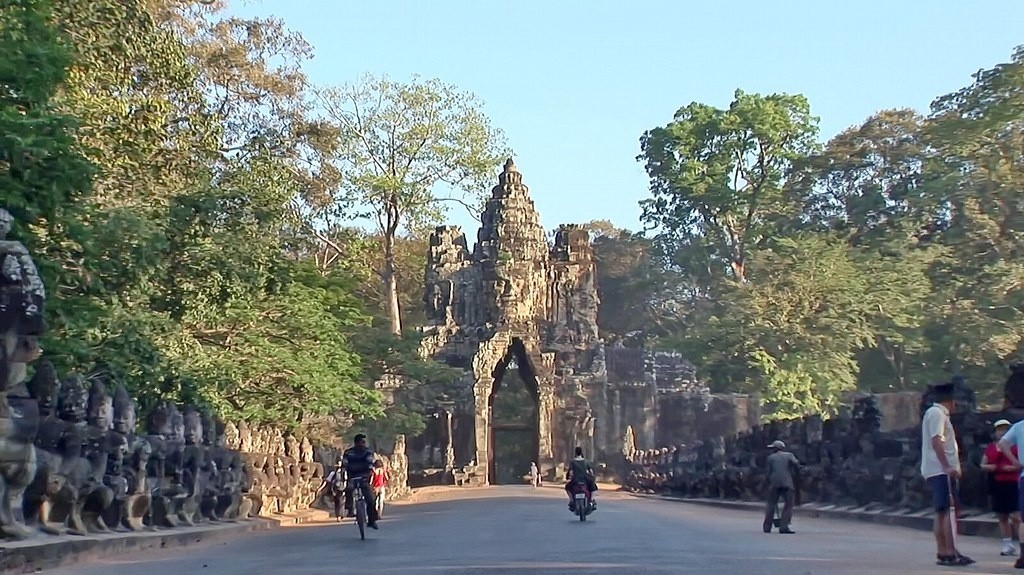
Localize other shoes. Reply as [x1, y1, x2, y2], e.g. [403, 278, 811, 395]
[567, 500, 575, 512]
[780, 527, 794, 533]
[936, 549, 975, 565]
[1000, 543, 1016, 556]
[588, 497, 597, 510]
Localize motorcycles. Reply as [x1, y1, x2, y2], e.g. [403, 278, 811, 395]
[567, 478, 597, 522]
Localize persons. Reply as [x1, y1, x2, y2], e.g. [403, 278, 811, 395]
[763, 440, 801, 534]
[920, 383, 974, 566]
[999, 418, 1024, 568]
[330, 459, 348, 522]
[565, 446, 597, 511]
[528, 462, 538, 488]
[369, 459, 387, 520]
[340, 434, 379, 529]
[980, 418, 1019, 556]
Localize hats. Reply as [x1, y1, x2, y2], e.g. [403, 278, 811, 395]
[929, 382, 966, 399]
[767, 440, 785, 449]
[994, 419, 1011, 427]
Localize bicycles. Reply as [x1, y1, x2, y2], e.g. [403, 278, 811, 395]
[343, 476, 372, 540]
